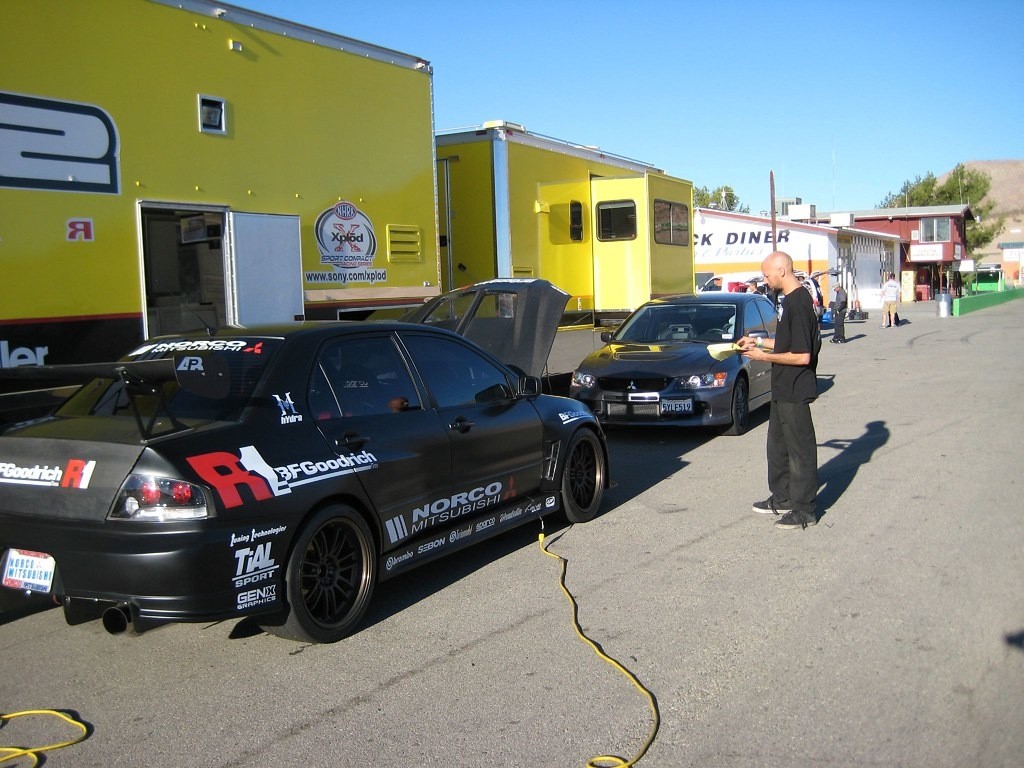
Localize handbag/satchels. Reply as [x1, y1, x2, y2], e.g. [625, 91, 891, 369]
[813, 304, 827, 318]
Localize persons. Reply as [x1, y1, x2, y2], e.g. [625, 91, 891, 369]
[748, 281, 763, 296]
[830, 281, 847, 343]
[879, 273, 901, 329]
[175, 267, 202, 303]
[812, 272, 825, 323]
[735, 252, 823, 529]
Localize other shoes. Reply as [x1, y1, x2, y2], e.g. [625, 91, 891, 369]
[830, 337, 839, 343]
[838, 336, 846, 343]
[881, 324, 887, 328]
[890, 325, 898, 328]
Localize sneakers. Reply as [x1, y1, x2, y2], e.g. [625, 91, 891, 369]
[752, 496, 792, 515]
[774, 509, 816, 530]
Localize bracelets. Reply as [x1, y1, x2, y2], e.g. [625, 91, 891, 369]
[756, 337, 762, 348]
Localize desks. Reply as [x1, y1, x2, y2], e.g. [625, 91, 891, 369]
[149, 306, 222, 335]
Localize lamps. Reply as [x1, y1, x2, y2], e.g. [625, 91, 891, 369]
[412, 62, 424, 70]
[214, 8, 226, 17]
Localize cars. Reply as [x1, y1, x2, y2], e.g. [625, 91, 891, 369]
[0, 276, 612, 645]
[569, 293, 777, 437]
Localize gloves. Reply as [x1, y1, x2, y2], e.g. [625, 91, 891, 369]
[814, 301, 818, 306]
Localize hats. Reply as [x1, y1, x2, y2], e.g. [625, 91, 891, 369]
[810, 271, 822, 278]
[831, 281, 839, 291]
[890, 273, 895, 277]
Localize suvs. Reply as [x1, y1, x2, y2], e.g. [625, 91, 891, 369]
[694, 267, 842, 325]
